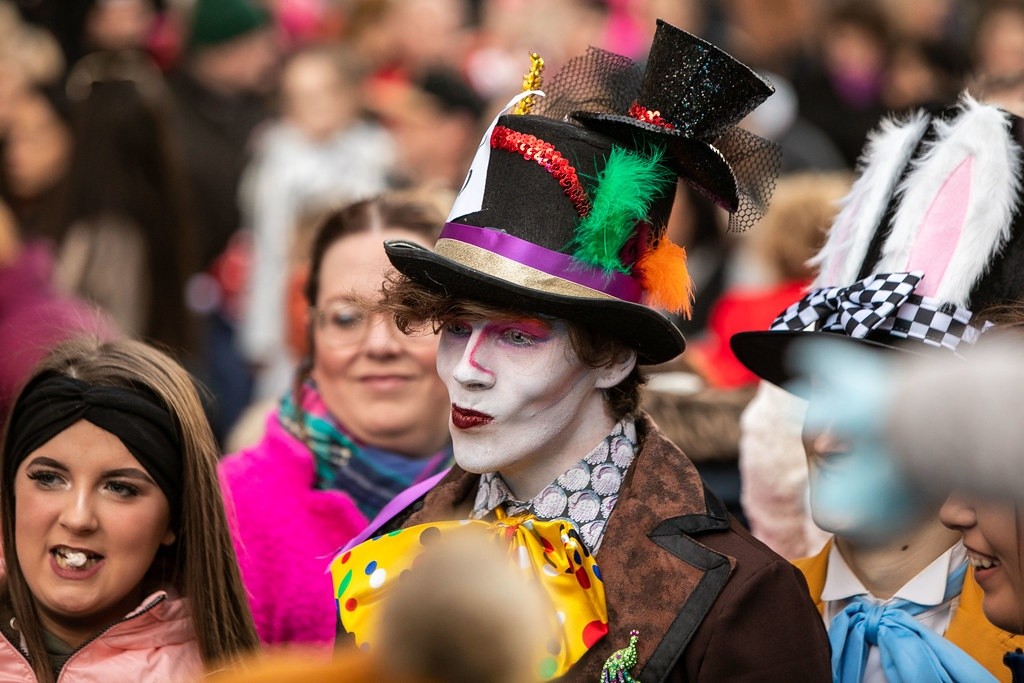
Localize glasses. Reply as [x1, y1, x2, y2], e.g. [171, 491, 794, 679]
[311, 294, 438, 349]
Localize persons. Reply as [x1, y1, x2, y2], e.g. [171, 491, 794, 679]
[216, 197, 456, 642]
[335, 113, 834, 682]
[0, 335, 259, 683]
[0, 1, 1023, 532]
[739, 103, 1024, 683]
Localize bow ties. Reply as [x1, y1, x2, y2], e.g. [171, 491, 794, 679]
[328, 509, 614, 678]
[829, 561, 1001, 681]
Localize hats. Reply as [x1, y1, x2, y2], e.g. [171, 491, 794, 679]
[386, 15, 779, 369]
[727, 95, 1024, 401]
